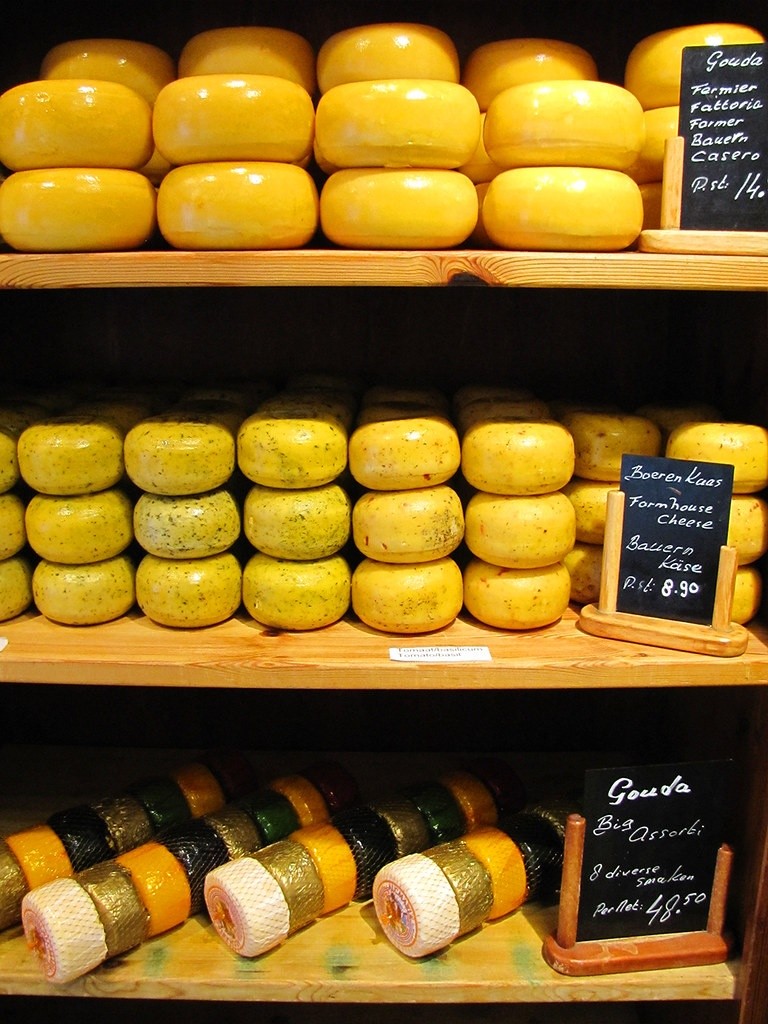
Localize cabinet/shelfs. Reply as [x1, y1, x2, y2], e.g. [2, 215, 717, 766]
[0, 246, 768, 1024]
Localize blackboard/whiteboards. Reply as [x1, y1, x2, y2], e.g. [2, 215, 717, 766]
[621, 453, 734, 626]
[679, 43, 768, 230]
[576, 761, 732, 943]
